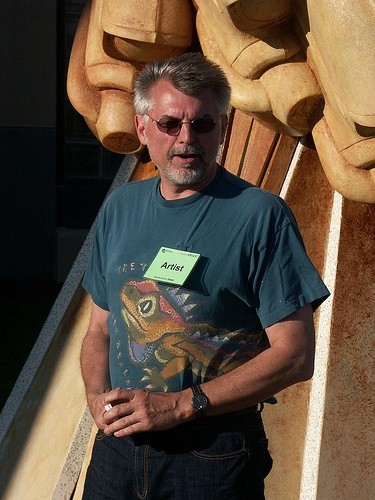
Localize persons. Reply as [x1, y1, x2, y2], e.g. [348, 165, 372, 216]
[79, 55, 330, 500]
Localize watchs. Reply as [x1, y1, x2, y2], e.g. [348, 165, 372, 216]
[190, 385, 212, 414]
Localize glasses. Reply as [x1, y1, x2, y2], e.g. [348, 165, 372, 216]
[145, 113, 220, 133]
